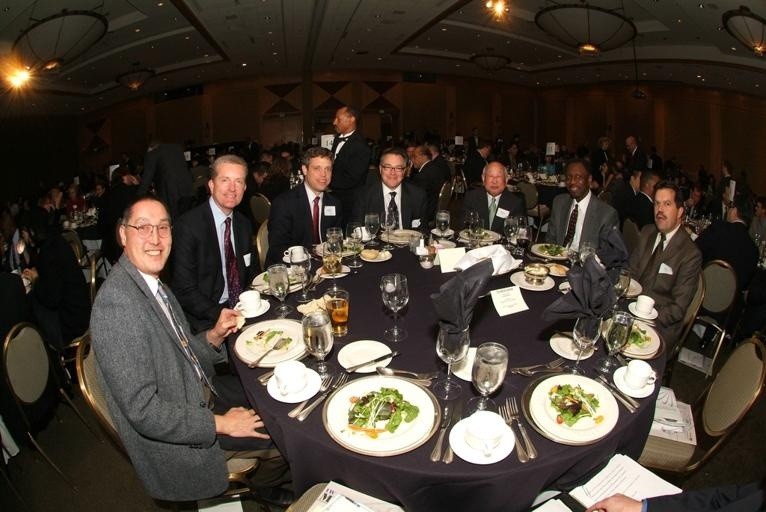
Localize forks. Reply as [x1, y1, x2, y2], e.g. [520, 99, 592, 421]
[498, 395, 538, 464]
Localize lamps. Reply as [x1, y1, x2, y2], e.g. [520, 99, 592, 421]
[467, 46, 511, 74]
[722, 5, 766, 58]
[10, 0, 110, 74]
[114, 60, 156, 94]
[532, 0, 638, 58]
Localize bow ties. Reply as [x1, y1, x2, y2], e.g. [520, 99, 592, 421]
[336, 137, 347, 142]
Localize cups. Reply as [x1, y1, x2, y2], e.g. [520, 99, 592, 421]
[63, 220, 77, 229]
[324, 289, 350, 338]
[464, 411, 504, 456]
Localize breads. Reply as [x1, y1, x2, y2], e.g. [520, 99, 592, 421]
[362, 248, 379, 260]
[550, 264, 568, 276]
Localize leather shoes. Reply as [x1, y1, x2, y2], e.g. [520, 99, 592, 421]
[257, 489, 296, 505]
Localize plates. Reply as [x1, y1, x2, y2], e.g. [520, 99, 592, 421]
[448, 414, 516, 464]
[231, 227, 503, 457]
[510, 241, 667, 446]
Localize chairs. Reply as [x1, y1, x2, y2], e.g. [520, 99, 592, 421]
[284, 481, 406, 512]
[623, 219, 766, 479]
[427, 151, 599, 227]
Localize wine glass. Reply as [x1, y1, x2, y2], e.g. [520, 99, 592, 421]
[436, 209, 532, 258]
[267, 247, 334, 377]
[289, 170, 302, 189]
[70, 210, 84, 225]
[379, 273, 509, 415]
[683, 208, 722, 234]
[322, 209, 397, 296]
[752, 232, 766, 269]
[561, 241, 634, 377]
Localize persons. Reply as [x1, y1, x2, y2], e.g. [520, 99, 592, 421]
[1, 105, 766, 511]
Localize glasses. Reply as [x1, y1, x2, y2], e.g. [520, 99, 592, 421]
[123, 222, 174, 240]
[380, 164, 407, 172]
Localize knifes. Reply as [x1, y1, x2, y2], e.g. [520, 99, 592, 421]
[430, 398, 465, 464]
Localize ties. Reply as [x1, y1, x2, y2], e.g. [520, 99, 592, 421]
[224, 217, 241, 307]
[488, 197, 496, 230]
[312, 196, 322, 245]
[157, 280, 221, 398]
[639, 233, 666, 293]
[563, 204, 578, 248]
[388, 192, 399, 230]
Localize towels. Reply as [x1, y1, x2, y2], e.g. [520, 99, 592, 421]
[428, 258, 500, 366]
[538, 254, 621, 344]
